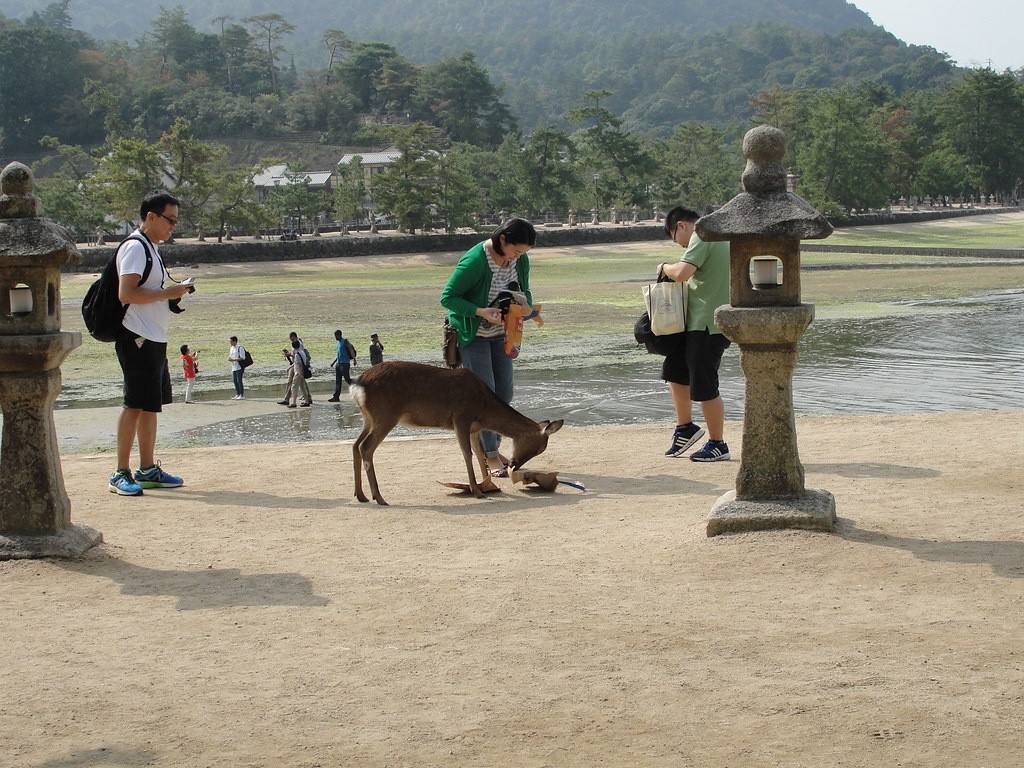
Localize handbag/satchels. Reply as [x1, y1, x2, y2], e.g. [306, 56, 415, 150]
[344, 338, 356, 360]
[504, 300, 523, 359]
[443, 317, 462, 368]
[642, 262, 689, 336]
[303, 366, 312, 379]
[237, 346, 254, 369]
[193, 361, 198, 373]
[633, 267, 688, 356]
[303, 349, 311, 367]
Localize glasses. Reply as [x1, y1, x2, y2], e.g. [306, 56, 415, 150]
[151, 209, 178, 225]
[674, 220, 682, 242]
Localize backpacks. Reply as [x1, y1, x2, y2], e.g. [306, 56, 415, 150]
[82, 237, 153, 343]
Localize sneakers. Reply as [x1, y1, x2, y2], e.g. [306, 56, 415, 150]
[134, 460, 183, 489]
[690, 439, 731, 461]
[664, 422, 706, 457]
[108, 470, 144, 496]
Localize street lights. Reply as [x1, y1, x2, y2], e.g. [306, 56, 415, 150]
[593, 174, 602, 222]
[273, 180, 282, 234]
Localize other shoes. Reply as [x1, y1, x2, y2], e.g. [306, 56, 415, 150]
[277, 400, 289, 405]
[301, 400, 312, 404]
[491, 467, 509, 477]
[185, 400, 194, 403]
[484, 458, 509, 468]
[231, 395, 238, 400]
[328, 396, 340, 402]
[287, 404, 296, 408]
[235, 395, 246, 400]
[300, 403, 309, 407]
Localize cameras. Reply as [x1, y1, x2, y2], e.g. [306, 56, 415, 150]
[181, 277, 196, 294]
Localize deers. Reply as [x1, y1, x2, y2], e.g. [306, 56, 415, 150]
[348, 360, 565, 507]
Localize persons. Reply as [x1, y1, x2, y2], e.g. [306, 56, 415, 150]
[440, 218, 537, 477]
[328, 330, 357, 402]
[656, 205, 731, 463]
[108, 191, 195, 496]
[369, 334, 385, 366]
[276, 332, 312, 408]
[181, 345, 198, 404]
[228, 337, 246, 400]
